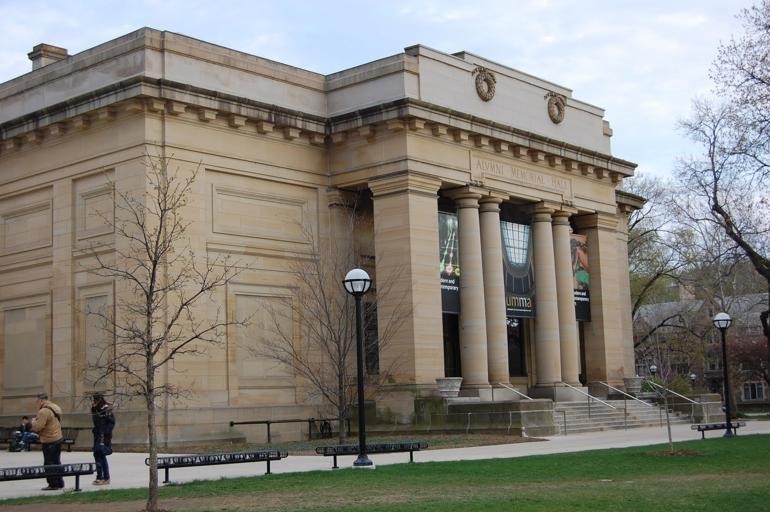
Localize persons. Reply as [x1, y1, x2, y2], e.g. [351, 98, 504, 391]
[8, 415, 39, 452]
[30, 391, 65, 491]
[89, 392, 116, 485]
[572, 242, 590, 290]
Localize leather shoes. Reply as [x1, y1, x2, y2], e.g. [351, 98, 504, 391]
[42, 486, 58, 490]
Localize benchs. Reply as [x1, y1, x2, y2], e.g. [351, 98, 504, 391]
[314, 439, 429, 469]
[691, 421, 746, 439]
[0, 461, 98, 491]
[8, 437, 75, 452]
[145, 450, 288, 483]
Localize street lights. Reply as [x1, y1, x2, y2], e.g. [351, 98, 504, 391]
[690, 373, 696, 390]
[711, 312, 736, 438]
[344, 266, 376, 468]
[649, 365, 659, 406]
